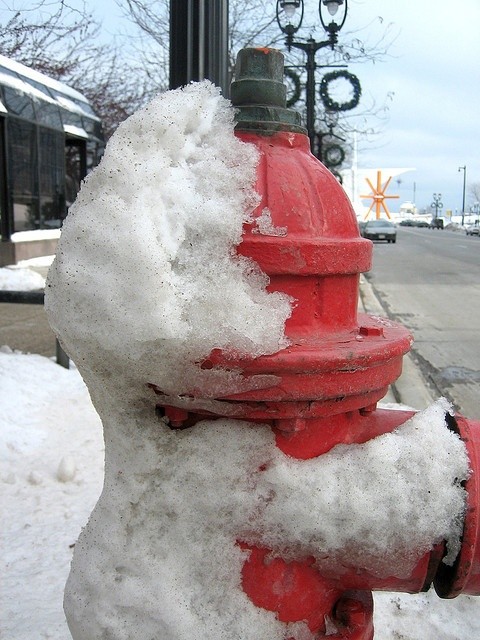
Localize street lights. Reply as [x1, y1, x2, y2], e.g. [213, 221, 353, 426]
[458, 162, 467, 224]
[277, 0, 347, 151]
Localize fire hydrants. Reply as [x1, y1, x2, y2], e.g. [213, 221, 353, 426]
[142, 46, 480, 640]
